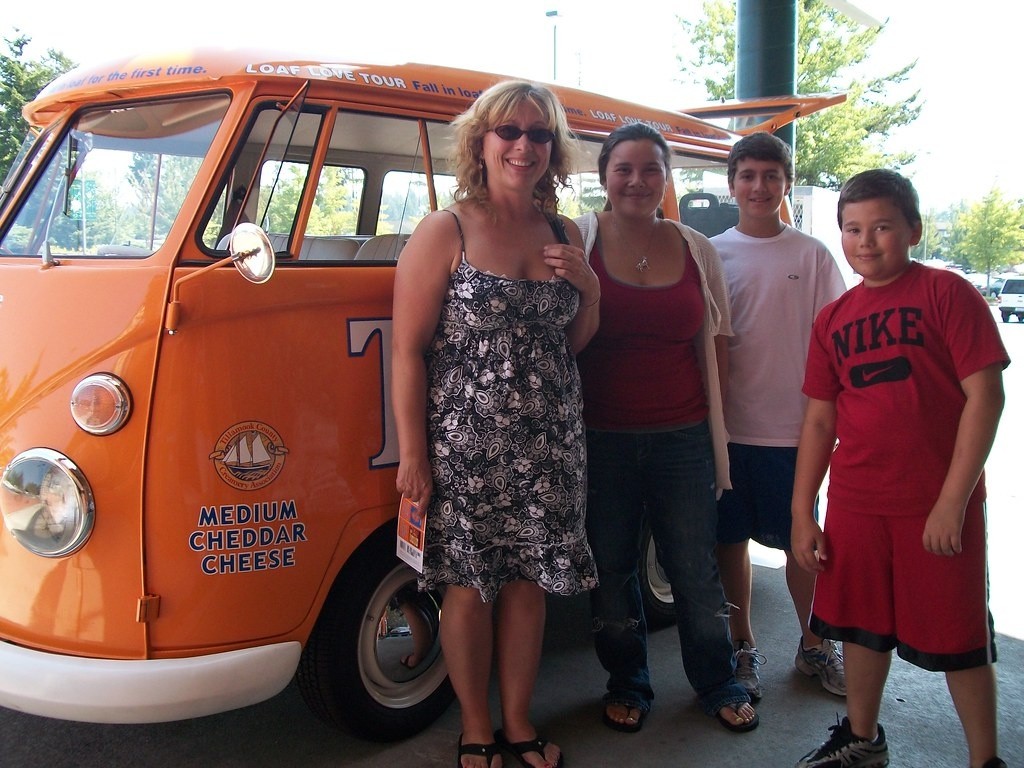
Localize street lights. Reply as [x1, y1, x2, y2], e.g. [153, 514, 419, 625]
[547, 7, 559, 81]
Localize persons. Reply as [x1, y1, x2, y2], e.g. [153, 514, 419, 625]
[693, 131, 849, 700]
[392, 81, 600, 768]
[573, 122, 761, 734]
[791, 169, 1011, 768]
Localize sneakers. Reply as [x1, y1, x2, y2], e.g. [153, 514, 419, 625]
[795, 635, 849, 696]
[983, 756, 1007, 768]
[733, 640, 768, 699]
[796, 713, 889, 768]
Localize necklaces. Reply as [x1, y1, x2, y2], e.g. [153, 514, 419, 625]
[610, 215, 657, 275]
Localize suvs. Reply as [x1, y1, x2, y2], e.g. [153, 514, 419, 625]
[998, 277, 1024, 323]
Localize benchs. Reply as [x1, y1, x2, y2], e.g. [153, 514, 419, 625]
[216, 232, 360, 261]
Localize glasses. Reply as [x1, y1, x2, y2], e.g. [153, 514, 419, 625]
[484, 124, 557, 145]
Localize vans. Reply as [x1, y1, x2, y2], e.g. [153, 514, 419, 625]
[0, 57, 848, 746]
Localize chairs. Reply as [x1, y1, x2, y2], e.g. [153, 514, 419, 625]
[354, 234, 410, 261]
[679, 192, 740, 238]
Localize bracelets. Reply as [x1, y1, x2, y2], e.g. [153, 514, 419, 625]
[580, 294, 601, 309]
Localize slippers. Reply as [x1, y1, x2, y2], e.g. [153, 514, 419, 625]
[457, 734, 504, 768]
[494, 728, 563, 768]
[603, 700, 645, 732]
[717, 704, 760, 732]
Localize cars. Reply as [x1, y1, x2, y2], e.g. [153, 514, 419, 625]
[973, 277, 1003, 297]
